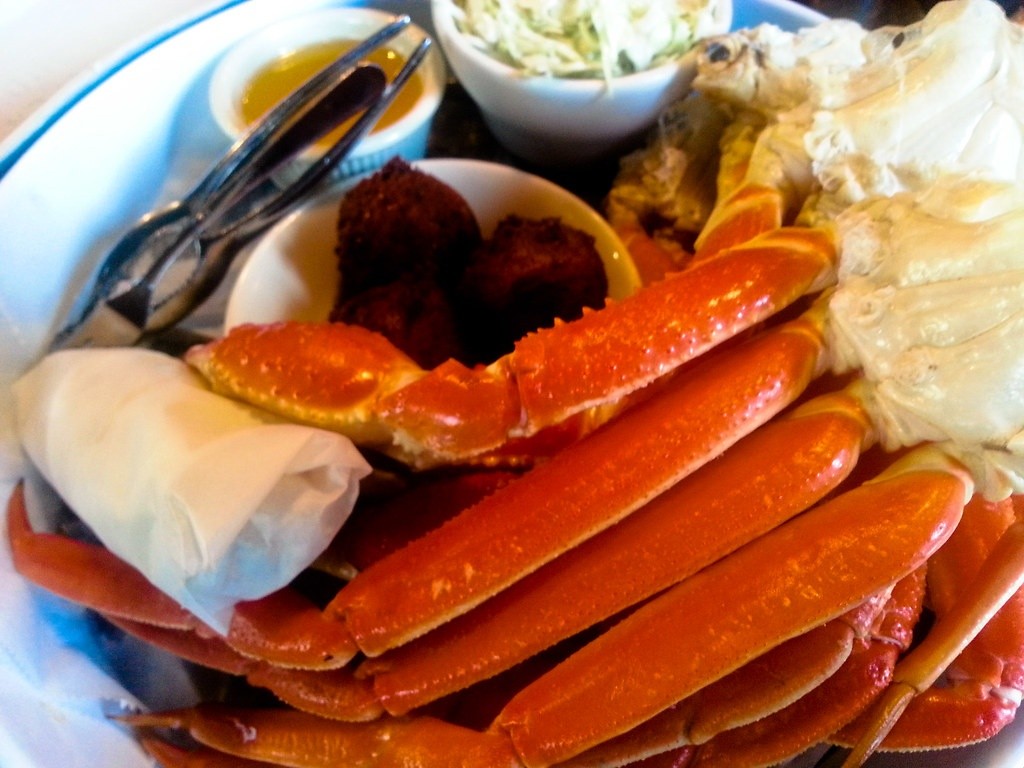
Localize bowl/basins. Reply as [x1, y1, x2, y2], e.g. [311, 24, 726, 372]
[207, 8, 448, 188]
[224, 159, 642, 340]
[429, 0, 734, 168]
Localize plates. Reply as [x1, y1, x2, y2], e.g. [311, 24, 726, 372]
[0, 0, 1024, 768]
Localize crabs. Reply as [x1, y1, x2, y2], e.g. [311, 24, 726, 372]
[7, 0, 1024, 768]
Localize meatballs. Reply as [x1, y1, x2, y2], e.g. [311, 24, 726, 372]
[321, 157, 607, 372]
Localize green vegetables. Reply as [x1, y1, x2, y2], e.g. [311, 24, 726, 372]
[449, 0, 725, 90]
